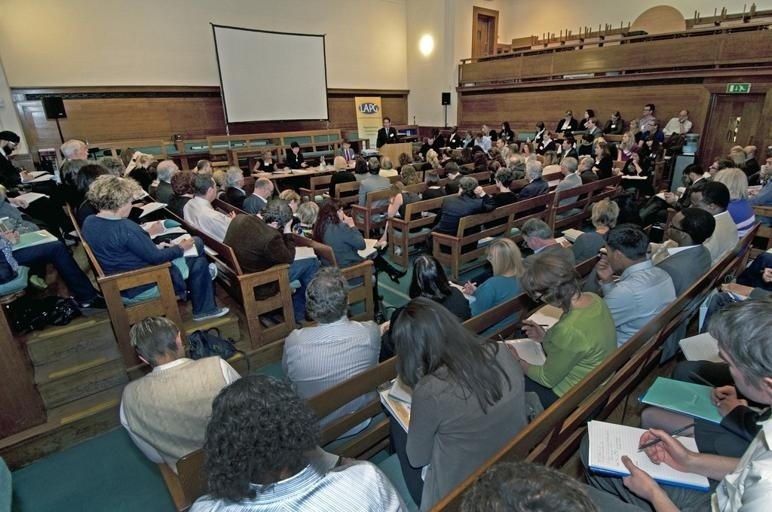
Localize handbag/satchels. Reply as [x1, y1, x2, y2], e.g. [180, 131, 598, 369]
[4, 293, 84, 337]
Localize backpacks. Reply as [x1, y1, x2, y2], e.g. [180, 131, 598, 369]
[189, 326, 250, 372]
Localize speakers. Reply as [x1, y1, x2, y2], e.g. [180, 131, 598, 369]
[442, 91, 452, 106]
[42, 97, 67, 120]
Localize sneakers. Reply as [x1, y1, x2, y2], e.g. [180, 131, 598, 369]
[208, 262, 218, 281]
[78, 295, 108, 309]
[64, 232, 81, 241]
[192, 306, 230, 321]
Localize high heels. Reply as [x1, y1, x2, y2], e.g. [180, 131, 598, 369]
[373, 240, 388, 252]
[373, 295, 383, 303]
[390, 268, 408, 285]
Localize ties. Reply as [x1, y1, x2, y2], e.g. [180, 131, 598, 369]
[345, 151, 350, 161]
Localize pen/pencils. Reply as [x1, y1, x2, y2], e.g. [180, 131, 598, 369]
[12, 228, 16, 234]
[499, 334, 510, 350]
[639, 423, 696, 449]
[529, 325, 549, 327]
[462, 282, 477, 289]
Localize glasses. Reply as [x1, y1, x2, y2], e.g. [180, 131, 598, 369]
[668, 223, 684, 232]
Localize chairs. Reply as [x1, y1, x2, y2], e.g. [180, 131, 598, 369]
[65, 125, 772, 512]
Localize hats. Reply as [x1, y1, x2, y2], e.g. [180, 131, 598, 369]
[0, 130, 21, 144]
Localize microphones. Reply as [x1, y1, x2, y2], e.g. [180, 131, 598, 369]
[413, 112, 416, 124]
